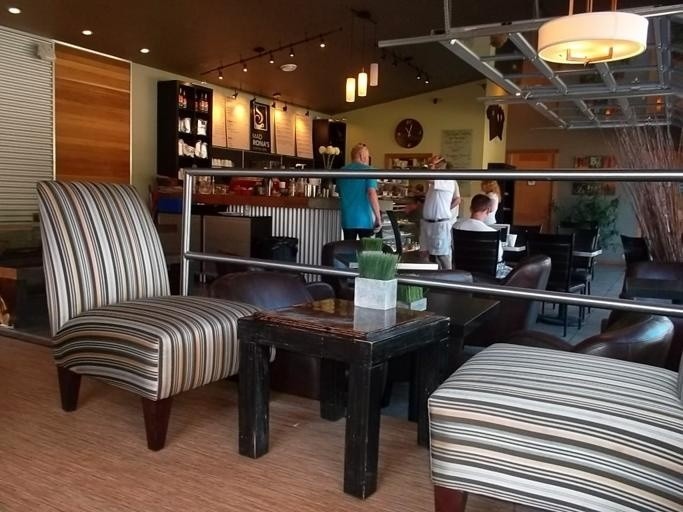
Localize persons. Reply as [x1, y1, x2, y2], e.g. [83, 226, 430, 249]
[445, 162, 460, 226]
[450, 194, 513, 279]
[480, 180, 501, 224]
[419, 153, 454, 270]
[335, 143, 382, 241]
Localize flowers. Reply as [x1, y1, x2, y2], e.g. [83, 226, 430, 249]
[317, 143, 340, 170]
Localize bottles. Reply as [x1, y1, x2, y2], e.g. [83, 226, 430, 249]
[179, 87, 187, 108]
[194, 92, 208, 114]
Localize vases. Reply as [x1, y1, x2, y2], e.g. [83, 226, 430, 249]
[321, 167, 332, 194]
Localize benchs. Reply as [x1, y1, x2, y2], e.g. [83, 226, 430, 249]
[0, 225, 42, 329]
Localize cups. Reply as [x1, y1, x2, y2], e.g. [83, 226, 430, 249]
[304, 184, 336, 198]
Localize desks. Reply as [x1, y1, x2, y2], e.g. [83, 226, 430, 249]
[236, 294, 450, 500]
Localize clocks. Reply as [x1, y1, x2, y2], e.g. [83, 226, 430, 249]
[393, 118, 423, 148]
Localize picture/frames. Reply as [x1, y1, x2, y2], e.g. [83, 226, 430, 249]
[570, 153, 617, 198]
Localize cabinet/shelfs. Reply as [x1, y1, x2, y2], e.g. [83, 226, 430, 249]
[157, 79, 211, 182]
[486, 159, 517, 228]
[312, 119, 344, 170]
[157, 213, 276, 295]
[377, 208, 432, 264]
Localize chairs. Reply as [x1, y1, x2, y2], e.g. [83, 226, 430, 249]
[320, 237, 397, 295]
[391, 269, 475, 297]
[453, 223, 600, 335]
[430, 344, 683, 511]
[619, 233, 652, 266]
[34, 179, 281, 452]
[387, 256, 551, 424]
[262, 235, 335, 301]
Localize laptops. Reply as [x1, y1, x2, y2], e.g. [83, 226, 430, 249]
[492, 224, 510, 246]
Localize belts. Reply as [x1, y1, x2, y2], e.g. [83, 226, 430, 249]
[419, 217, 450, 223]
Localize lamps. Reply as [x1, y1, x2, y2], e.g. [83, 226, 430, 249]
[33, 42, 56, 61]
[341, 7, 379, 107]
[535, 1, 647, 68]
[230, 85, 310, 117]
[197, 26, 341, 82]
[378, 45, 433, 90]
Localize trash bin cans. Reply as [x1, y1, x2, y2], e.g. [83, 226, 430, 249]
[265, 237, 298, 262]
